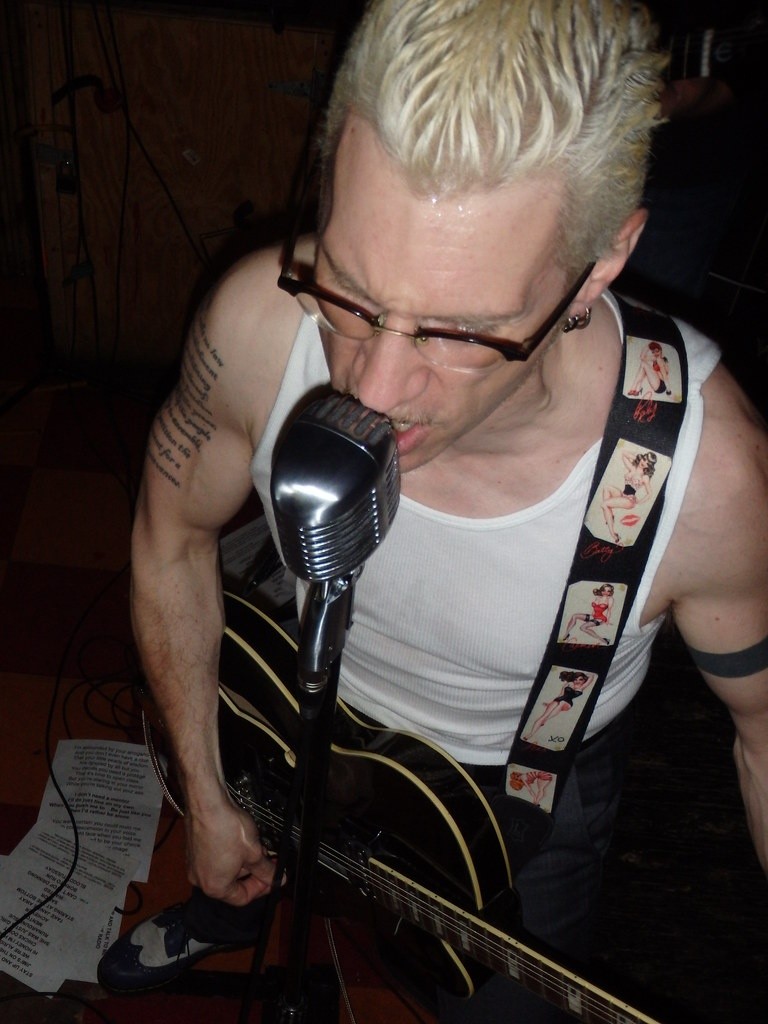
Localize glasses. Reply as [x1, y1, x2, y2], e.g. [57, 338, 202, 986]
[276, 220, 596, 377]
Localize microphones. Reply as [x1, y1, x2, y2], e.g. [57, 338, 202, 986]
[268, 388, 401, 721]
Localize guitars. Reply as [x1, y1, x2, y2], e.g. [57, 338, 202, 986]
[139, 573, 664, 1023]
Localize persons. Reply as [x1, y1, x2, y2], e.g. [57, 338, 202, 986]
[97, 1, 768, 1024]
